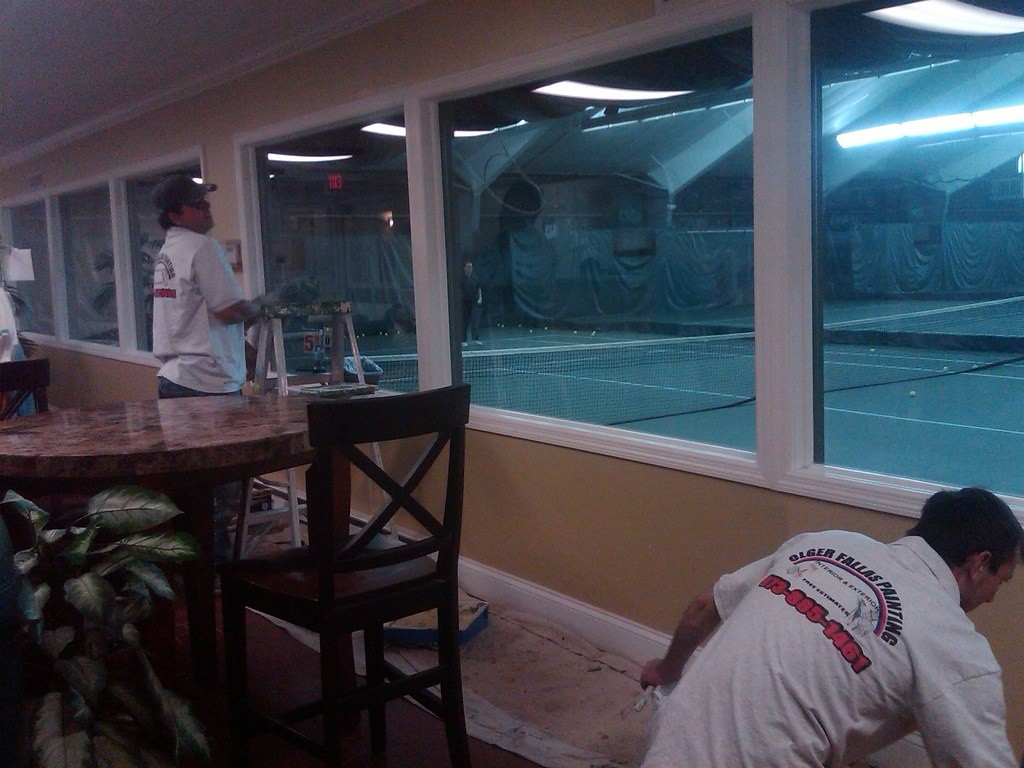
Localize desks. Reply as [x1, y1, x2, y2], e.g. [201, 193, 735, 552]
[0, 388, 409, 677]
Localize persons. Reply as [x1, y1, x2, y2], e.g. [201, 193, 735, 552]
[460, 261, 482, 347]
[639, 487, 1024, 768]
[0, 284, 34, 416]
[279, 285, 326, 372]
[152, 175, 271, 566]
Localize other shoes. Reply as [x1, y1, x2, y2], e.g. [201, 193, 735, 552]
[472, 340, 483, 346]
[461, 341, 468, 346]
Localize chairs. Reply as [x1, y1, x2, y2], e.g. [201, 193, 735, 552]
[218, 383, 472, 768]
[0, 358, 50, 420]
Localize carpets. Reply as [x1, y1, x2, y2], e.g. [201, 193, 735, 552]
[166, 531, 658, 768]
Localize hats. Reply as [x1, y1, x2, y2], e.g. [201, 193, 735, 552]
[153, 173, 217, 209]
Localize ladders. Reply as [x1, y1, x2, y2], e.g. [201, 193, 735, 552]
[232, 302, 402, 558]
